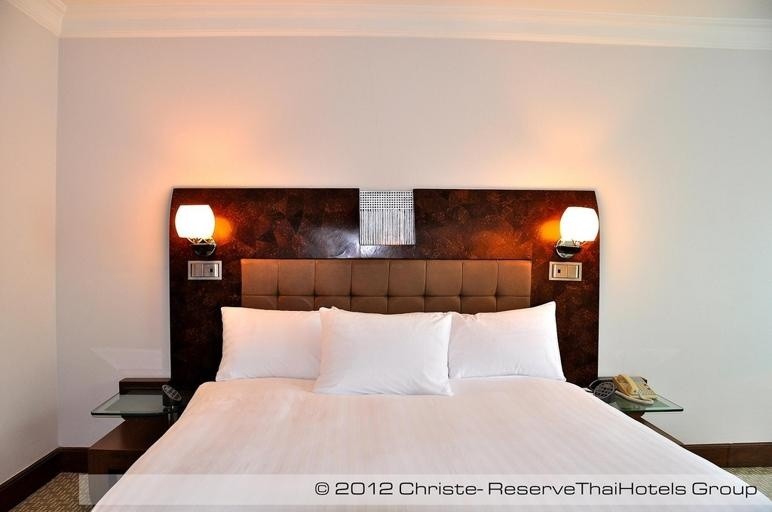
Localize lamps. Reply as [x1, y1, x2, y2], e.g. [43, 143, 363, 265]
[555, 206, 599, 258]
[175, 205, 217, 257]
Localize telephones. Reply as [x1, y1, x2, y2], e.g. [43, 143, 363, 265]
[612, 373, 658, 400]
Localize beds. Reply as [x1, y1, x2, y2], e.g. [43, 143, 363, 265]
[91, 188, 772, 512]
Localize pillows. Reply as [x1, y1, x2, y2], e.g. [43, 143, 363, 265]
[215, 306, 320, 380]
[312, 307, 454, 400]
[447, 302, 568, 381]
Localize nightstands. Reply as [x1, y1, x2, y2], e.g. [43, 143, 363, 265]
[87, 378, 185, 504]
[606, 393, 684, 449]
